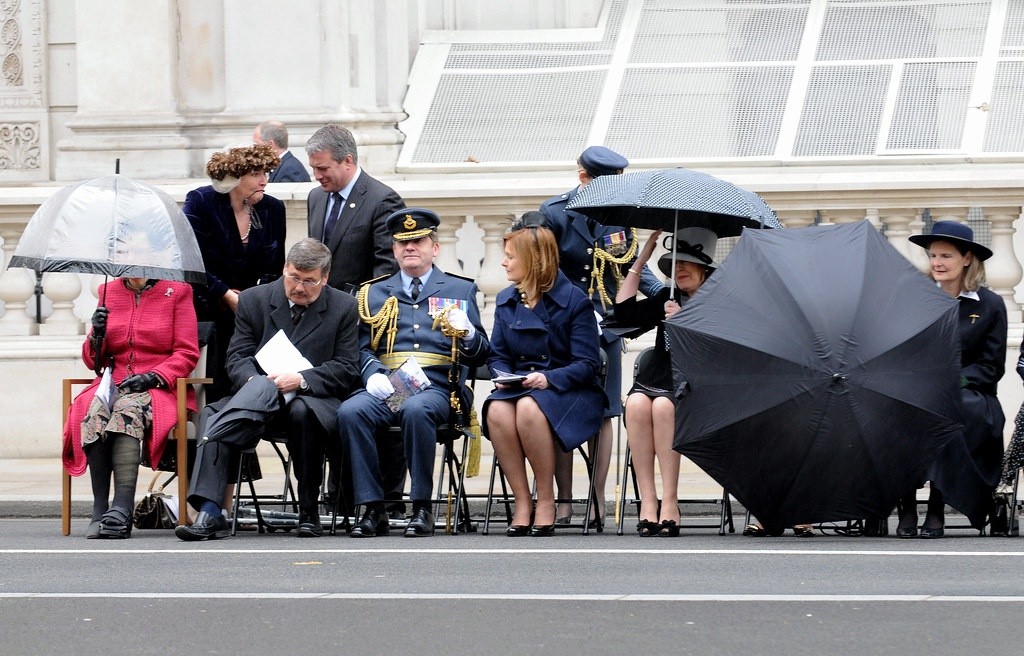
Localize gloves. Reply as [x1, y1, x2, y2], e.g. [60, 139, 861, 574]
[448, 309, 476, 340]
[91, 306, 110, 339]
[119, 371, 159, 393]
[366, 372, 395, 399]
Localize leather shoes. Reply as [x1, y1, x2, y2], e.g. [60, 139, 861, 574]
[404, 507, 436, 537]
[298, 514, 323, 537]
[175, 511, 230, 539]
[350, 505, 390, 537]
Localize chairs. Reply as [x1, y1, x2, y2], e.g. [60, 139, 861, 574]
[617, 347, 734, 535]
[355, 379, 475, 537]
[231, 434, 352, 536]
[62, 321, 214, 536]
[482, 346, 610, 535]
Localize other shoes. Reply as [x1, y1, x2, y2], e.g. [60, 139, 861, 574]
[555, 510, 574, 523]
[920, 516, 945, 537]
[100, 502, 132, 538]
[85, 520, 109, 539]
[583, 514, 604, 527]
[895, 515, 918, 537]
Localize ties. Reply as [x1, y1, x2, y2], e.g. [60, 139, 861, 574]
[411, 277, 422, 301]
[587, 217, 595, 237]
[324, 192, 344, 243]
[292, 304, 307, 325]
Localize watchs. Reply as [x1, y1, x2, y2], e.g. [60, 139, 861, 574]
[299, 375, 309, 391]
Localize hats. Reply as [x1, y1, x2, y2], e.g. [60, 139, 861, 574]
[577, 145, 629, 179]
[908, 220, 993, 263]
[658, 226, 720, 280]
[510, 211, 549, 231]
[385, 208, 441, 241]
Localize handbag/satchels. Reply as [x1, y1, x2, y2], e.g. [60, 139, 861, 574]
[133, 470, 179, 529]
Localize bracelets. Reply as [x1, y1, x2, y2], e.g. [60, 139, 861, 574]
[627, 269, 643, 278]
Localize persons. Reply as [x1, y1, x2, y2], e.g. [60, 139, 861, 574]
[252, 120, 312, 183]
[334, 206, 491, 536]
[173, 237, 362, 540]
[137, 139, 287, 531]
[539, 146, 666, 530]
[61, 222, 200, 540]
[613, 227, 719, 537]
[743, 519, 816, 537]
[481, 210, 610, 537]
[896, 221, 1008, 539]
[304, 123, 410, 519]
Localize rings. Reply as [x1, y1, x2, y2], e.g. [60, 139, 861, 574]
[533, 386, 536, 389]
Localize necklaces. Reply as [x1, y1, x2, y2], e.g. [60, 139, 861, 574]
[241, 208, 253, 241]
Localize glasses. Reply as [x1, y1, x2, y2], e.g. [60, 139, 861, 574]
[282, 264, 321, 287]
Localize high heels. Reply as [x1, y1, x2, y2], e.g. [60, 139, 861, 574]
[636, 501, 661, 537]
[530, 508, 557, 536]
[658, 506, 681, 537]
[506, 503, 536, 536]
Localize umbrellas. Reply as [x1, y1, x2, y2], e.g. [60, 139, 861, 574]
[6, 158, 211, 378]
[661, 220, 966, 537]
[599, 296, 656, 341]
[196, 375, 286, 467]
[563, 166, 785, 301]
